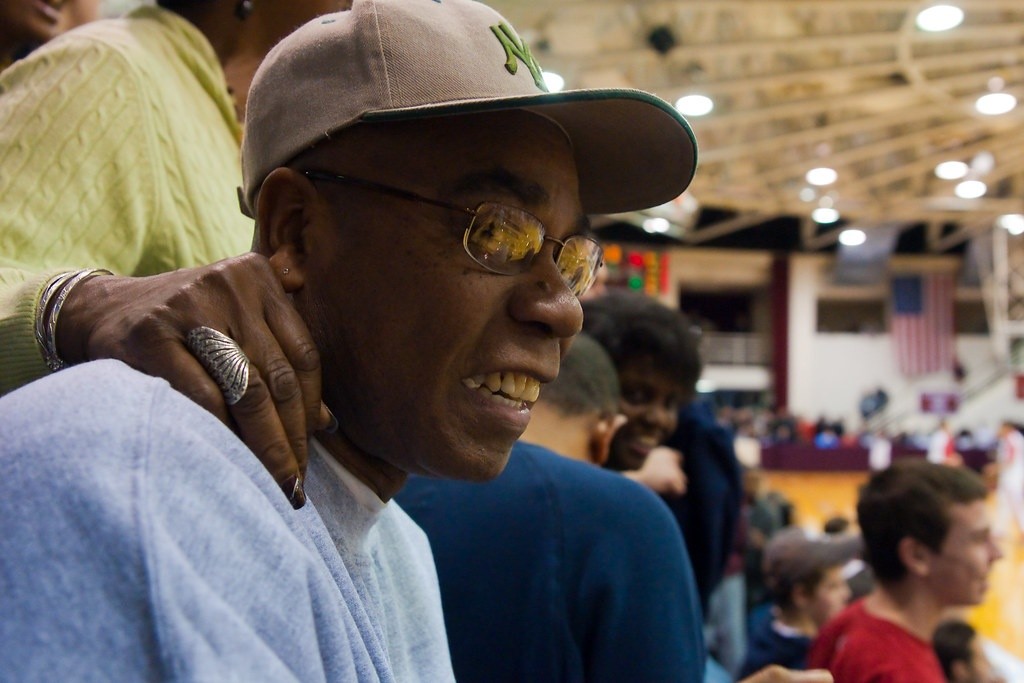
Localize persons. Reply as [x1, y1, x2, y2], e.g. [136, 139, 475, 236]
[661, 375, 1023, 682]
[388, 331, 708, 683]
[0, 0, 833, 681]
[566, 287, 705, 499]
[0, 1, 356, 512]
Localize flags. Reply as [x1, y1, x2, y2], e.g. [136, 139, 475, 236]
[879, 269, 958, 375]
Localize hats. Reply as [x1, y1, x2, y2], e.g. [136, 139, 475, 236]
[233, 1, 699, 220]
[762, 525, 865, 594]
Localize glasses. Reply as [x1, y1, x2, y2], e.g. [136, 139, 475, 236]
[304, 165, 604, 300]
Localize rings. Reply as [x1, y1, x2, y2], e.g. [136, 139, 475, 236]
[185, 324, 251, 406]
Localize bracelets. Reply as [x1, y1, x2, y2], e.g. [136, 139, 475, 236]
[33, 267, 117, 372]
